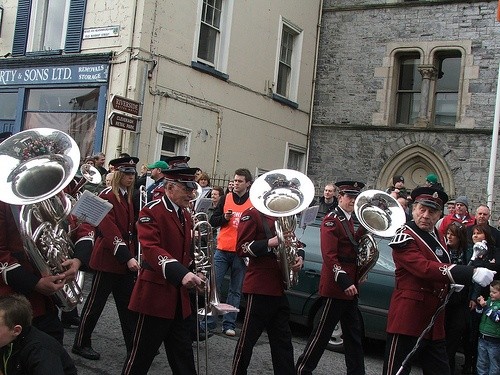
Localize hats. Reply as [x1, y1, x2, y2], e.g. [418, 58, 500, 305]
[426, 173, 438, 184]
[335, 180, 365, 195]
[166, 156, 191, 169]
[410, 187, 448, 209]
[454, 196, 469, 208]
[162, 167, 198, 189]
[109, 157, 139, 173]
[145, 161, 169, 171]
[392, 175, 404, 185]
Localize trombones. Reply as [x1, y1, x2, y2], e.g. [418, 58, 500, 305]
[194, 212, 220, 375]
[138, 185, 148, 278]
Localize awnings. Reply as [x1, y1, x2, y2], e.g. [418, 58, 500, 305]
[69, 88, 100, 104]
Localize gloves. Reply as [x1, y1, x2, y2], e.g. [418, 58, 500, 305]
[450, 284, 464, 292]
[472, 268, 497, 287]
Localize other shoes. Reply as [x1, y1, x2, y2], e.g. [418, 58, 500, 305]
[194, 333, 214, 341]
[62, 315, 81, 328]
[221, 327, 235, 336]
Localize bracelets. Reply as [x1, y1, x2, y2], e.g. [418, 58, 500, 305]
[299, 256, 304, 269]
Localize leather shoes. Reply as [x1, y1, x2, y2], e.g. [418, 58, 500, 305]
[73, 347, 100, 358]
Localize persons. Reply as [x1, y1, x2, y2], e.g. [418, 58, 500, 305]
[0, 294, 77, 375]
[193, 169, 254, 340]
[121, 166, 206, 375]
[383, 174, 448, 219]
[0, 132, 191, 359]
[295, 180, 368, 375]
[434, 195, 500, 375]
[381, 187, 497, 375]
[235, 203, 306, 375]
[318, 183, 338, 218]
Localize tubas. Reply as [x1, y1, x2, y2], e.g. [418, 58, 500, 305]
[353, 189, 406, 285]
[248, 168, 315, 289]
[0, 127, 84, 309]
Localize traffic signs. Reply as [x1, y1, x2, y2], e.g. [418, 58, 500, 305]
[107, 111, 137, 133]
[111, 94, 139, 116]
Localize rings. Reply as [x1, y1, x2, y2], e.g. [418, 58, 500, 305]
[191, 286, 193, 288]
[194, 284, 197, 287]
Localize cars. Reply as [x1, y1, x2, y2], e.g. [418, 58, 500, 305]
[212, 216, 399, 353]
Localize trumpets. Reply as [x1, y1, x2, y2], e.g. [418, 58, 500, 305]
[69, 163, 101, 194]
